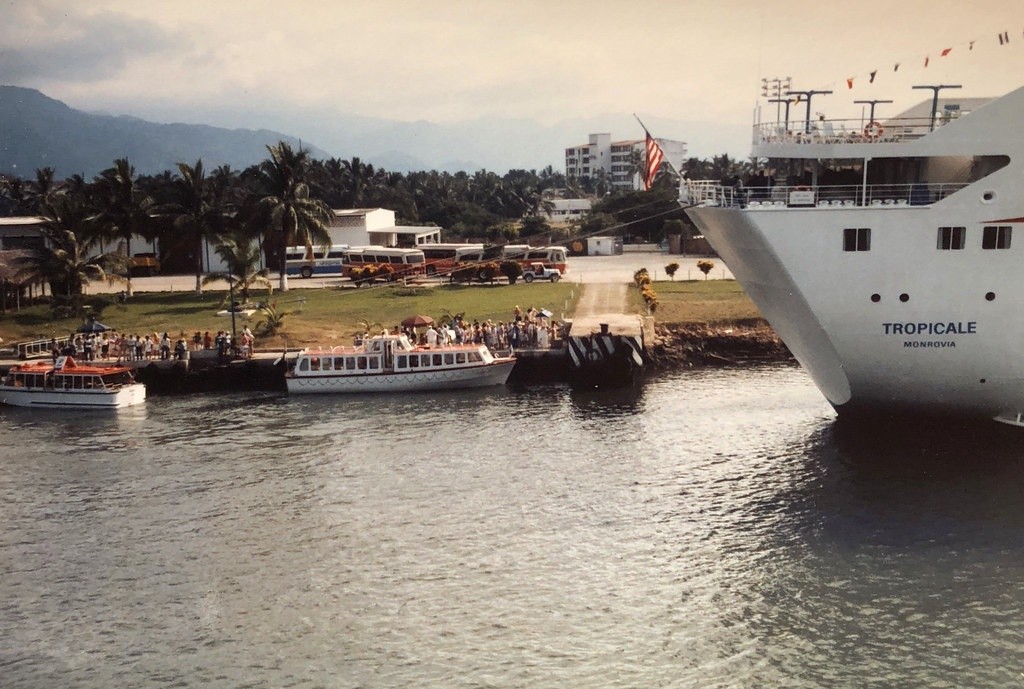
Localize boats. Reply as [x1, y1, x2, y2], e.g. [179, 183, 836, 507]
[0, 357, 146, 409]
[284, 328, 518, 394]
[678, 78, 1024, 418]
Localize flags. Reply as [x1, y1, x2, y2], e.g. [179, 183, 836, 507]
[643, 132, 664, 191]
[793, 33, 1008, 105]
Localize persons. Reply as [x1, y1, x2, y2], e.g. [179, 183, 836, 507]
[391, 305, 537, 348]
[51, 325, 255, 363]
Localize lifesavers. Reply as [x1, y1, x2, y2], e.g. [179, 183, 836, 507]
[864, 122, 883, 139]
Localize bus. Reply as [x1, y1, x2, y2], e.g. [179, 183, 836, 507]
[417, 244, 484, 275]
[286, 244, 350, 278]
[455, 246, 567, 282]
[343, 248, 426, 281]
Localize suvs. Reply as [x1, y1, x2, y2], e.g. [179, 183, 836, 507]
[522, 262, 563, 283]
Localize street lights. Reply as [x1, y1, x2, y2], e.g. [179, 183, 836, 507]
[225, 245, 236, 334]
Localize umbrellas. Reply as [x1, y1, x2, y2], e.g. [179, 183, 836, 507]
[76, 322, 110, 337]
[401, 315, 433, 335]
